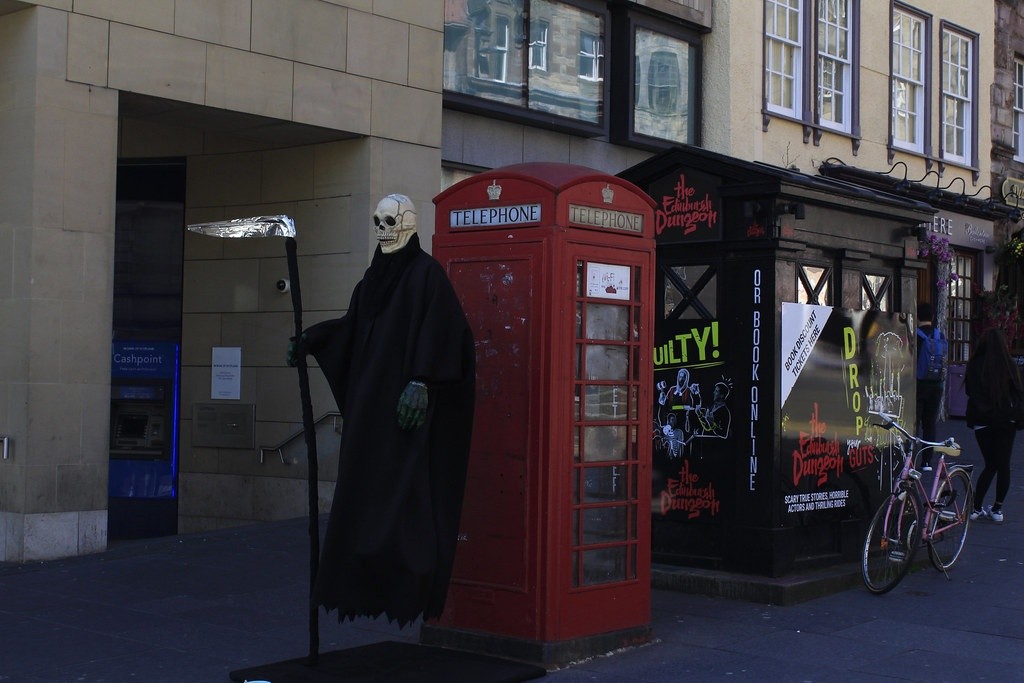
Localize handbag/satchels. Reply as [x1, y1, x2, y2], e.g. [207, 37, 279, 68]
[1007, 389, 1024, 431]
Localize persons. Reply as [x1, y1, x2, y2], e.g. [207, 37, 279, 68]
[659, 370, 732, 439]
[917, 303, 945, 471]
[964, 328, 1024, 521]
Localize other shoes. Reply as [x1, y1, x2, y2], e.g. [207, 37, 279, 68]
[920, 462, 933, 471]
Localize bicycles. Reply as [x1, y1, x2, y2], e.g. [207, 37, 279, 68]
[860, 410, 977, 595]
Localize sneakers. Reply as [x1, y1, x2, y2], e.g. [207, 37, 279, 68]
[970, 506, 987, 520]
[987, 505, 1003, 521]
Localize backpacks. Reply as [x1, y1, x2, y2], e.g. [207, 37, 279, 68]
[917, 328, 947, 382]
